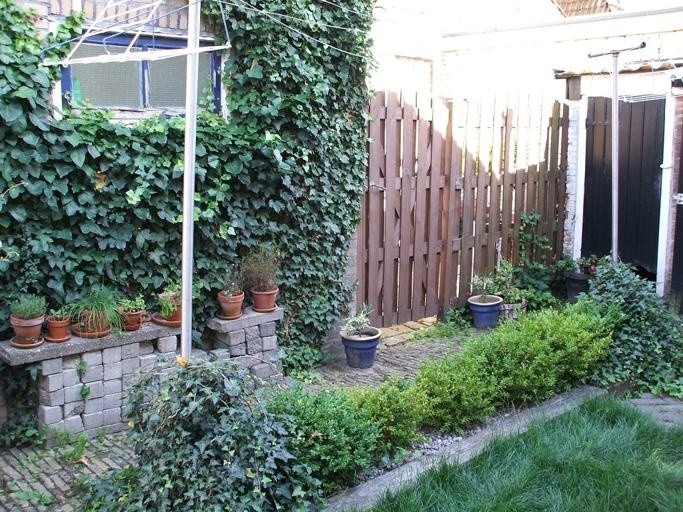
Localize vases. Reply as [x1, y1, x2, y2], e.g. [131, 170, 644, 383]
[564, 272, 594, 303]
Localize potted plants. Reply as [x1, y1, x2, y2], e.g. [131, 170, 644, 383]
[8, 278, 182, 349]
[339, 302, 383, 368]
[466, 260, 535, 330]
[216, 240, 285, 319]
